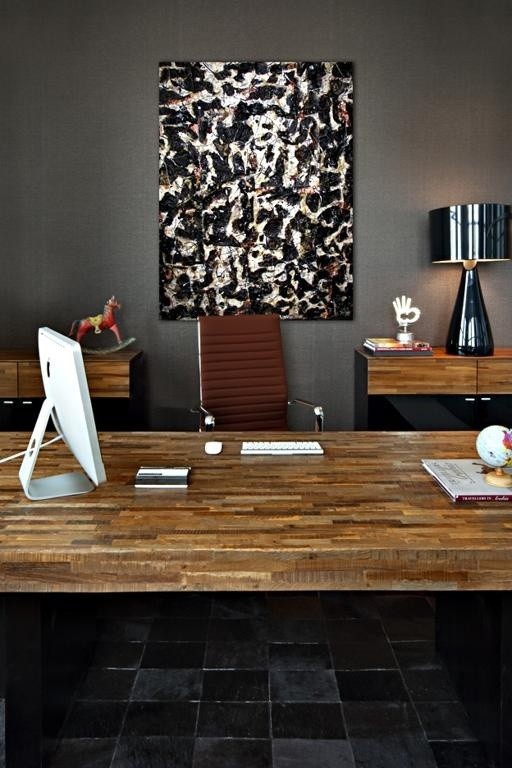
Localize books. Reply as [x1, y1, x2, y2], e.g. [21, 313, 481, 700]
[134, 464, 192, 490]
[365, 336, 412, 347]
[363, 342, 433, 351]
[420, 454, 512, 502]
[362, 346, 434, 358]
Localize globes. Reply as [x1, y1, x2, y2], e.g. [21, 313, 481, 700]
[476, 425, 512, 488]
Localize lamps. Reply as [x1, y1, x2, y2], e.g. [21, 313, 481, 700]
[429, 202, 512, 358]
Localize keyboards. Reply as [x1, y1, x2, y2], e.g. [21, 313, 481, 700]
[240, 441, 324, 455]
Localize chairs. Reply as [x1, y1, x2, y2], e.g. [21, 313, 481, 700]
[190, 316, 323, 431]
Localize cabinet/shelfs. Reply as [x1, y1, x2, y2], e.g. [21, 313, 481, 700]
[355, 348, 512, 431]
[1, 348, 145, 431]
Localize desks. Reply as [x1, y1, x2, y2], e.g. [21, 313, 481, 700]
[1, 432, 512, 767]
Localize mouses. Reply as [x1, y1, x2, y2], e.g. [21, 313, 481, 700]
[204, 441, 223, 454]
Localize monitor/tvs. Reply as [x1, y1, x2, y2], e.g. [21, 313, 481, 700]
[18, 326, 108, 502]
